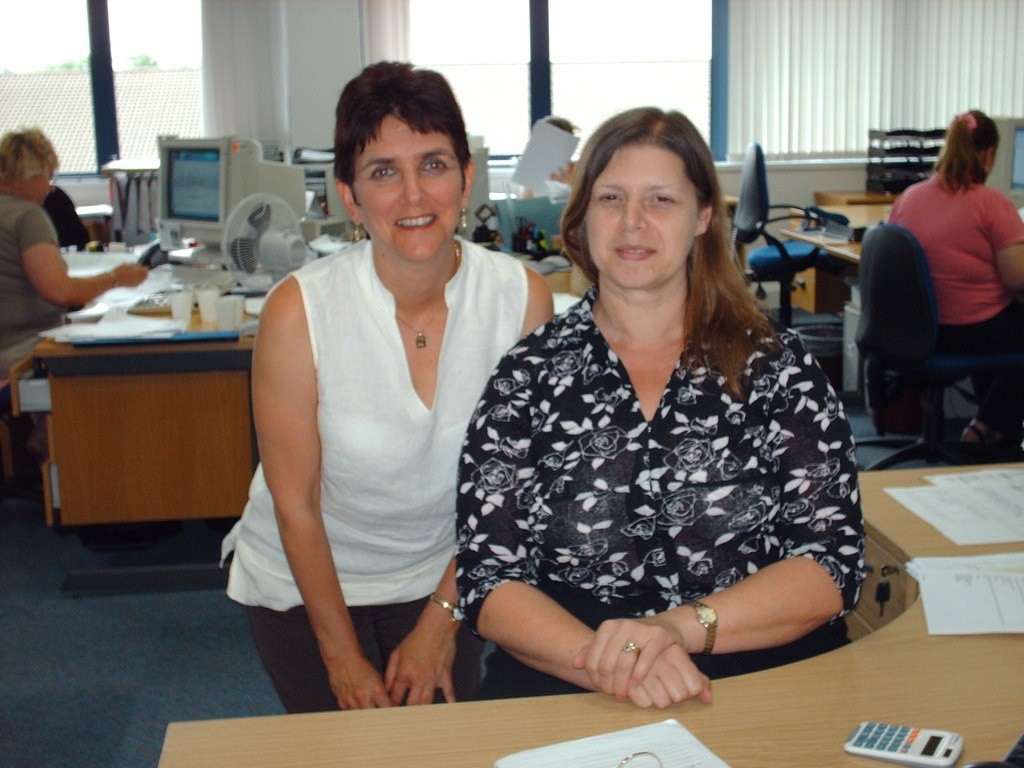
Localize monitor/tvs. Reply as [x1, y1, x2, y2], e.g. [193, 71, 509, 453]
[159, 137, 307, 262]
[1003, 119, 1024, 196]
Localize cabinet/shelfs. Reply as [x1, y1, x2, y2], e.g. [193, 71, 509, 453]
[790, 203, 895, 314]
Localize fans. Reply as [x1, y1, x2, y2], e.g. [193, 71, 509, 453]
[221, 192, 308, 337]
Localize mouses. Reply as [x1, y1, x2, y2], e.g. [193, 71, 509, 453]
[542, 255, 569, 268]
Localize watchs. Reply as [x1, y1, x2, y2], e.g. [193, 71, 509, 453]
[687, 600, 718, 654]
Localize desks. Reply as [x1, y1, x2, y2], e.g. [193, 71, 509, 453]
[812, 191, 899, 206]
[8, 262, 260, 599]
[779, 225, 862, 264]
[158, 461, 1024, 768]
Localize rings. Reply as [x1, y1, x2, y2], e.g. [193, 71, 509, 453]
[621, 641, 642, 655]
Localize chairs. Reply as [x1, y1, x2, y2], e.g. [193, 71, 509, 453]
[731, 143, 843, 331]
[853, 223, 1024, 470]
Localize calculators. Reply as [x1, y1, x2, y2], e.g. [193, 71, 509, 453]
[843, 720, 964, 767]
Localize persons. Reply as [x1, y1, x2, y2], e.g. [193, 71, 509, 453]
[1, 127, 149, 457]
[454, 106, 866, 710]
[522, 114, 581, 198]
[218, 61, 554, 713]
[888, 110, 1024, 450]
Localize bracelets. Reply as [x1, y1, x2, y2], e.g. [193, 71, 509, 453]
[105, 272, 116, 287]
[429, 592, 465, 622]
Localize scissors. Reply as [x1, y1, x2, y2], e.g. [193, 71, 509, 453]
[515, 216, 527, 231]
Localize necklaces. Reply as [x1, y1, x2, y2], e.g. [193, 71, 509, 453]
[395, 241, 461, 348]
